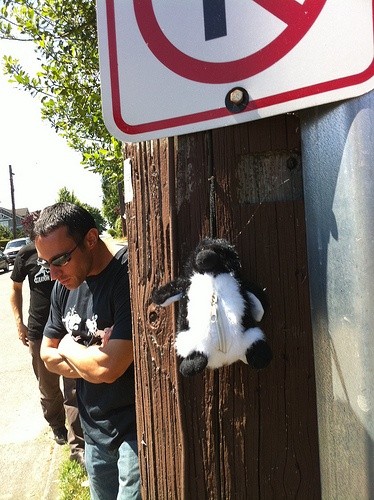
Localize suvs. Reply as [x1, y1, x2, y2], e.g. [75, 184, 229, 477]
[3, 237, 34, 265]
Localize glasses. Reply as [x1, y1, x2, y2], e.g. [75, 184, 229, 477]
[37, 237, 85, 268]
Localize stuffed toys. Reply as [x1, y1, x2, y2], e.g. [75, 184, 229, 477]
[153, 237, 270, 377]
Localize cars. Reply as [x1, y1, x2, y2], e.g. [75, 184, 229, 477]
[0, 250, 9, 272]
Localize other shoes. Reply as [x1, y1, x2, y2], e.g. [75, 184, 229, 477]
[70, 450, 88, 477]
[53, 430, 68, 445]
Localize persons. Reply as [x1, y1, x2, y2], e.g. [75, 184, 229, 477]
[29, 202, 142, 500]
[10, 242, 85, 468]
